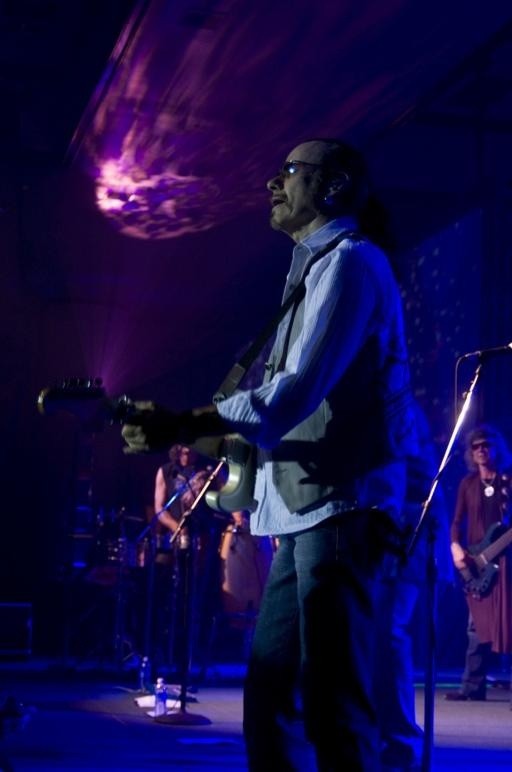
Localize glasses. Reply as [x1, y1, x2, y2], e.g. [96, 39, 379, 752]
[471, 442, 487, 450]
[280, 158, 322, 179]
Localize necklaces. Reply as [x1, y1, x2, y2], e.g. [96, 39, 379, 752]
[481, 473, 496, 497]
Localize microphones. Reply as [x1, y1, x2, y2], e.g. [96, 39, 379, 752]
[464, 340, 512, 360]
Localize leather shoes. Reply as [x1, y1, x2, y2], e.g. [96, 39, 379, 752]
[444, 688, 490, 704]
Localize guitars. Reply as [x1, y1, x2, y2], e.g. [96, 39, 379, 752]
[38, 375, 259, 513]
[455, 521, 510, 600]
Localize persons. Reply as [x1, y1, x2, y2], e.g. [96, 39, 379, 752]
[153, 445, 246, 550]
[445, 427, 512, 701]
[121, 138, 452, 772]
